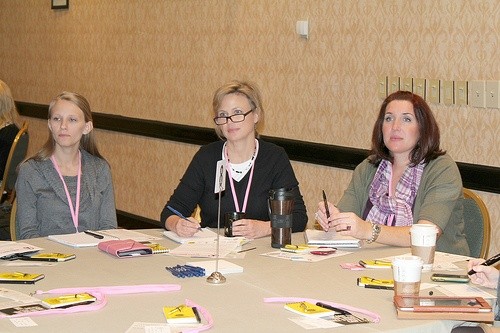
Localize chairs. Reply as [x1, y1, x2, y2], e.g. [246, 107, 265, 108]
[435, 188, 491, 260]
[0, 120, 30, 241]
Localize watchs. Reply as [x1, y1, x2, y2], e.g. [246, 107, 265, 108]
[366, 220, 382, 245]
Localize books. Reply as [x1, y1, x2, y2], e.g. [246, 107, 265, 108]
[47, 225, 163, 249]
[0, 244, 395, 324]
[304, 228, 362, 249]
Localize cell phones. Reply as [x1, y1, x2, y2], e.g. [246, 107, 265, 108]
[431, 273, 470, 282]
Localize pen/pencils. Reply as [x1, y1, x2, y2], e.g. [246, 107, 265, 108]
[0, 280, 34, 284]
[365, 285, 395, 290]
[84, 231, 104, 239]
[166, 206, 203, 231]
[19, 258, 58, 262]
[323, 190, 331, 228]
[192, 307, 201, 323]
[55, 300, 95, 309]
[165, 264, 206, 278]
[316, 303, 352, 315]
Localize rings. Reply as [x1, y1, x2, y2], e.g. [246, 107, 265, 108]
[346, 225, 352, 230]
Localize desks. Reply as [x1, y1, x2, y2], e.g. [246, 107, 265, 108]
[0, 228, 500, 333]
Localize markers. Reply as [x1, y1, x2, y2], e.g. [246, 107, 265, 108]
[467, 254, 500, 275]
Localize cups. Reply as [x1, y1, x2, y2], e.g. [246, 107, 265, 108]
[391, 256, 424, 296]
[266, 188, 297, 248]
[223, 212, 246, 237]
[409, 223, 440, 271]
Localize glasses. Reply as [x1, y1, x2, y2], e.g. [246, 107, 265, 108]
[213, 109, 253, 125]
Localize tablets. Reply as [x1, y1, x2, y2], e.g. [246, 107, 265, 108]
[394, 297, 492, 312]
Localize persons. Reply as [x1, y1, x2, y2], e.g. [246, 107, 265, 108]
[313, 90, 472, 257]
[13, 90, 119, 240]
[159, 79, 309, 239]
[0, 78, 28, 206]
[467, 259, 500, 289]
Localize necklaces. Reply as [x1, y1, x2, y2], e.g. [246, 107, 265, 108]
[226, 144, 257, 175]
